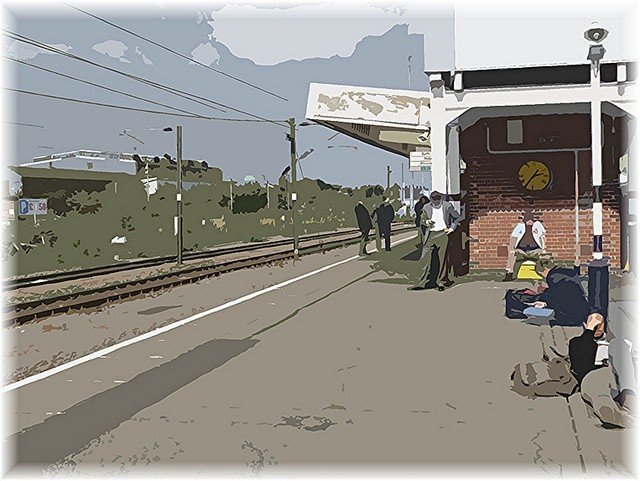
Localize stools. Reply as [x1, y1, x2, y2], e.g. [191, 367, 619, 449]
[517, 259, 542, 279]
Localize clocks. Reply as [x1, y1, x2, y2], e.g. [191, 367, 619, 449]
[516, 160, 551, 191]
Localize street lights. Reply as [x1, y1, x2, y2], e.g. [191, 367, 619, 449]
[584, 22, 609, 332]
